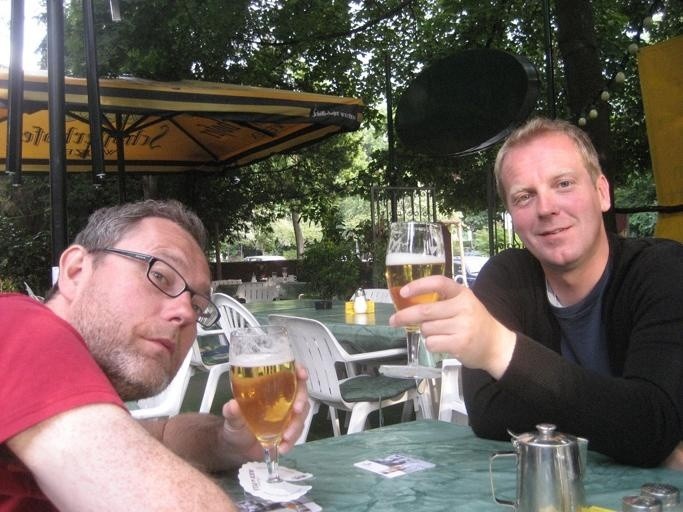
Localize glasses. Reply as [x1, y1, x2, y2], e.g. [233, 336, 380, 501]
[88, 246, 220, 328]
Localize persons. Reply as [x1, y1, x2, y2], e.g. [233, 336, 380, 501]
[0, 198, 312, 512]
[388, 116, 683, 469]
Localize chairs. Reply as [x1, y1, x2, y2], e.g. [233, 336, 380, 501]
[125, 279, 469, 445]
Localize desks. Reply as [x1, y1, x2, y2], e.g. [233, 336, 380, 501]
[208, 416, 682, 511]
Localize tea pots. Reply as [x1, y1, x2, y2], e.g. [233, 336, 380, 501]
[486, 420, 588, 512]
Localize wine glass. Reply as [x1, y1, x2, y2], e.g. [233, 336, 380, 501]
[377, 219, 442, 380]
[226, 328, 310, 497]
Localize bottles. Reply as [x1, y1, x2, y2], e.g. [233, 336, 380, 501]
[354, 288, 367, 313]
[623, 481, 679, 512]
